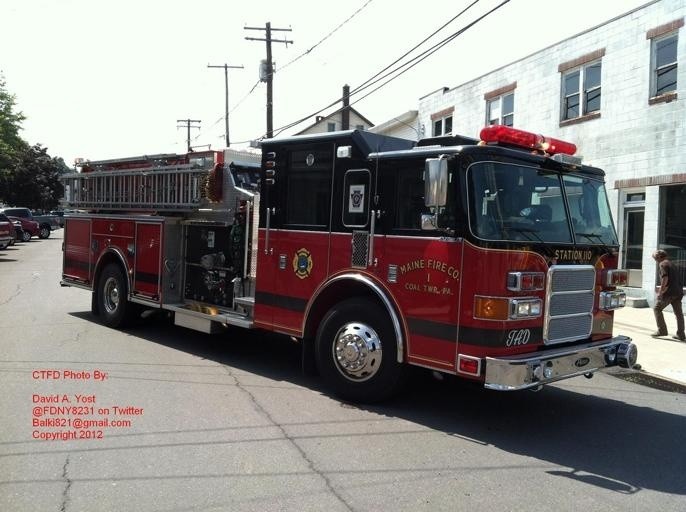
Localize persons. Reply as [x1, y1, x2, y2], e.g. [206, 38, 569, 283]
[648, 248, 686, 344]
[493, 166, 532, 218]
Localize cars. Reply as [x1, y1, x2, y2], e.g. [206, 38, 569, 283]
[0, 213, 40, 250]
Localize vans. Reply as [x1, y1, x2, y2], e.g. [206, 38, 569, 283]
[46, 211, 65, 228]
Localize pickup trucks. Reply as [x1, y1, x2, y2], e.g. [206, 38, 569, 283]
[1, 207, 60, 239]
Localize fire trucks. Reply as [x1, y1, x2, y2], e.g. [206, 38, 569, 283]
[57, 124, 638, 406]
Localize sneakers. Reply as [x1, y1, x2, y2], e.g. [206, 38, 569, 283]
[651, 330, 686, 341]
